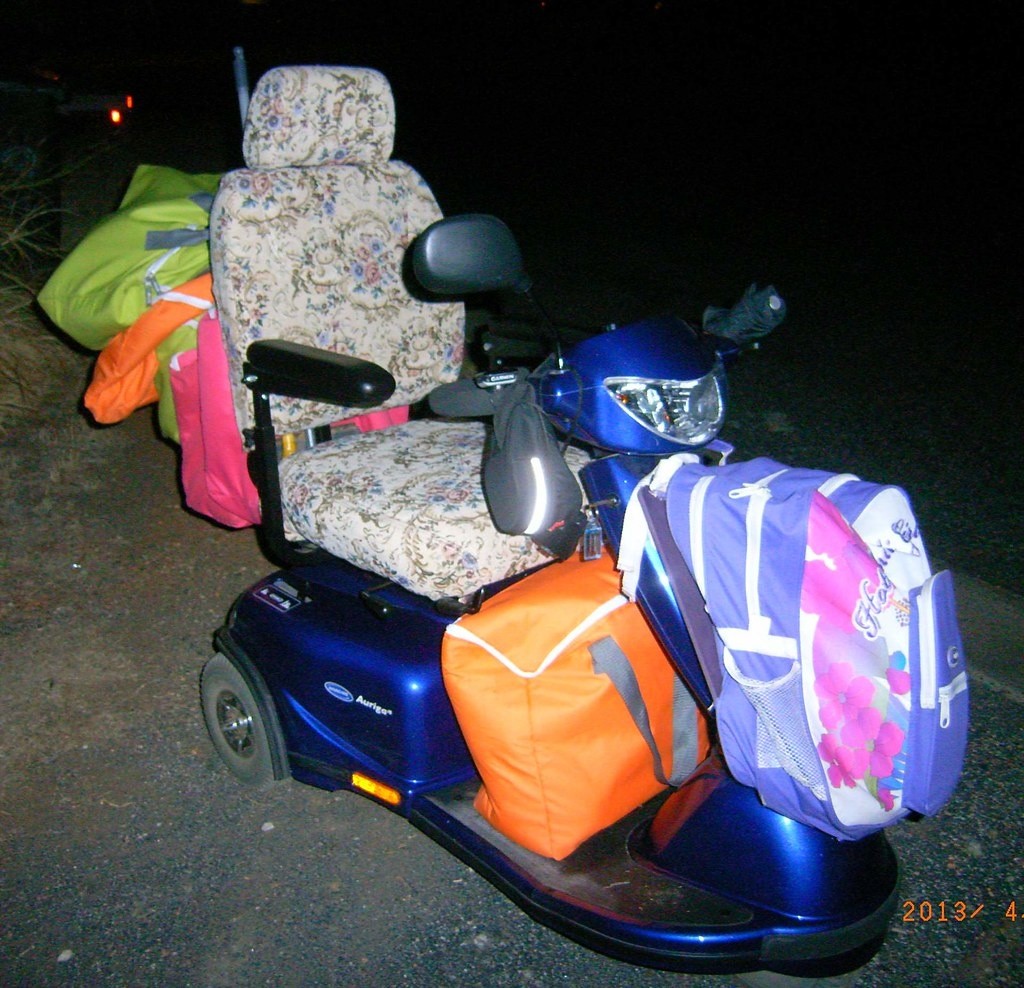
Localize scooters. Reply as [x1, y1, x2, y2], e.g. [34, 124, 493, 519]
[197, 66, 905, 976]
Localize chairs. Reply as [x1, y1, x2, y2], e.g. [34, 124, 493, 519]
[208, 64, 593, 599]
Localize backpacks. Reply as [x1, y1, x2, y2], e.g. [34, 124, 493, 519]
[638, 456, 970, 841]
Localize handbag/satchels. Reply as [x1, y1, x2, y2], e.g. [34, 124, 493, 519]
[36, 162, 411, 529]
[439, 548, 710, 861]
